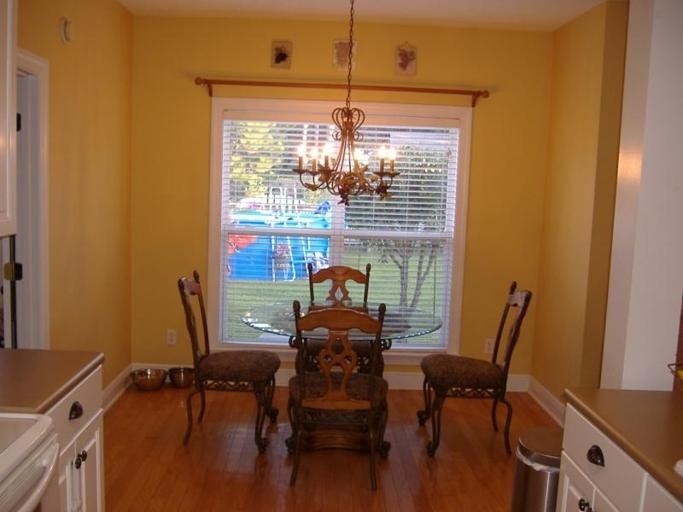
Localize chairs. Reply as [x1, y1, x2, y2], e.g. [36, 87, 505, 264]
[294, 263, 386, 375]
[415, 281, 532, 459]
[285, 299, 390, 493]
[178, 270, 281, 453]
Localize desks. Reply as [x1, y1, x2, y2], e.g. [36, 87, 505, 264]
[240, 302, 443, 462]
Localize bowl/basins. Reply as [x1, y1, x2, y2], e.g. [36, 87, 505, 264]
[168, 366, 194, 388]
[128, 368, 165, 391]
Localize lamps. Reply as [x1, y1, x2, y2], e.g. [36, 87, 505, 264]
[292, 1, 400, 206]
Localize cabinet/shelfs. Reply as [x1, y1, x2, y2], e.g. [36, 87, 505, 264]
[36, 357, 106, 512]
[555, 387, 682, 512]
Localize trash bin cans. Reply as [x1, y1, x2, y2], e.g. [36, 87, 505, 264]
[510, 424, 565, 511]
